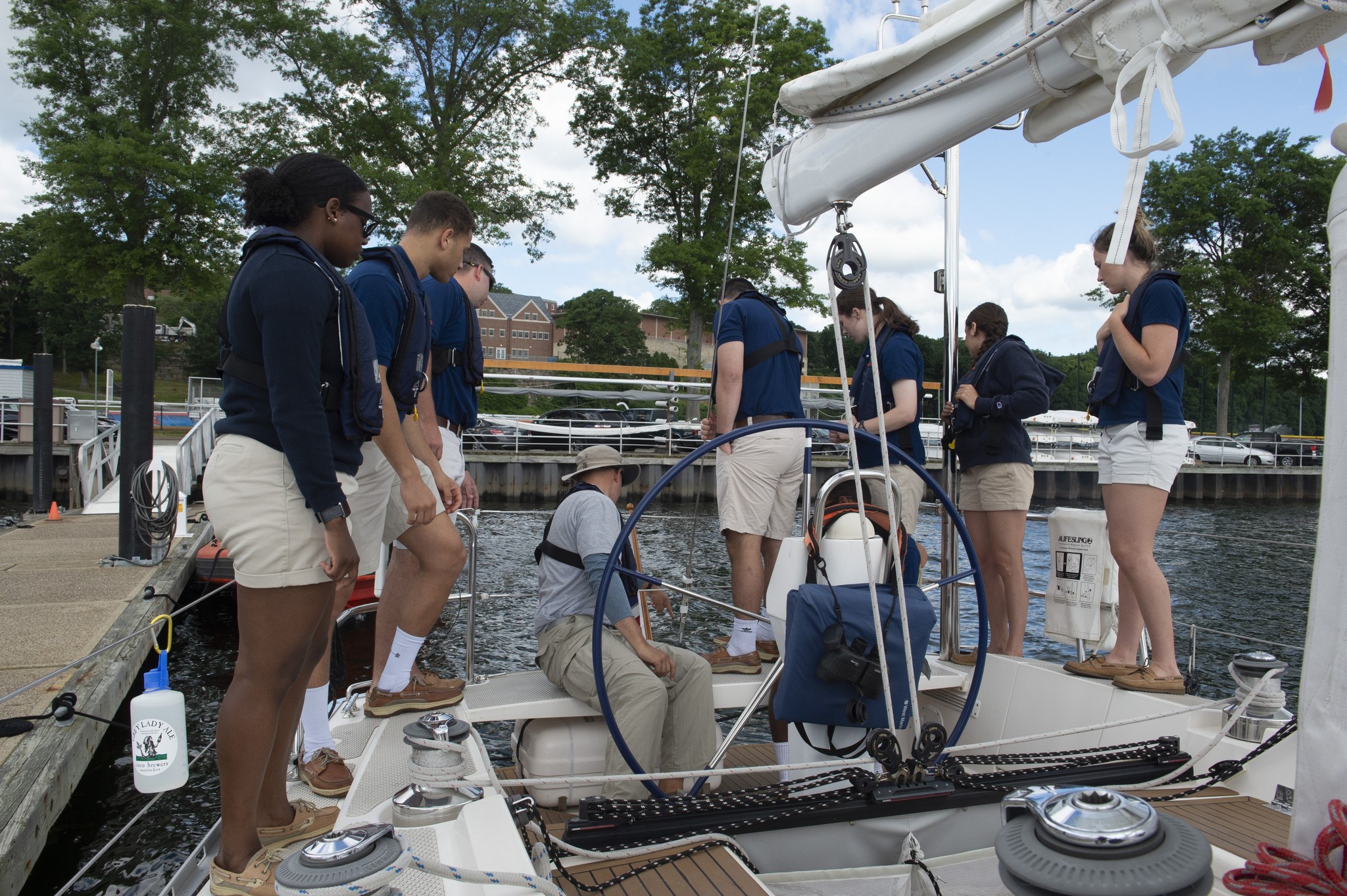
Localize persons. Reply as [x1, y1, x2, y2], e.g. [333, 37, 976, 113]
[698, 278, 806, 674]
[202, 153, 384, 896]
[532, 444, 717, 801]
[365, 243, 497, 701]
[827, 283, 927, 534]
[1063, 201, 1191, 696]
[818, 470, 927, 586]
[292, 191, 464, 797]
[940, 302, 1068, 665]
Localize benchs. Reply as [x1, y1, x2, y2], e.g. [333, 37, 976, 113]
[1130, 787, 1289, 861]
[457, 652, 958, 727]
[558, 846, 775, 895]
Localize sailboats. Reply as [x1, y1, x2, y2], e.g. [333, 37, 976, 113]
[149, 0, 1347, 896]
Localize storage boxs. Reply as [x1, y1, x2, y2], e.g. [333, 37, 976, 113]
[509, 718, 726, 806]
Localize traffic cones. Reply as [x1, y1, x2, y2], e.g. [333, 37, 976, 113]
[45, 501, 64, 520]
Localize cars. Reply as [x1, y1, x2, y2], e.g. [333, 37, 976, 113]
[811, 428, 851, 456]
[527, 407, 633, 452]
[1186, 435, 1276, 466]
[462, 413, 530, 450]
[1281, 439, 1324, 466]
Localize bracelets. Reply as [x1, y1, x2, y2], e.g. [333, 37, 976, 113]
[854, 423, 858, 427]
[644, 583, 653, 590]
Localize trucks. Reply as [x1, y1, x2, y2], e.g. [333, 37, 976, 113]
[154, 315, 198, 342]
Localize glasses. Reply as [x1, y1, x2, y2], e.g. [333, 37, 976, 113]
[458, 260, 496, 292]
[316, 200, 380, 239]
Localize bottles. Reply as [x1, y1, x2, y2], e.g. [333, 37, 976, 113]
[130, 649, 189, 794]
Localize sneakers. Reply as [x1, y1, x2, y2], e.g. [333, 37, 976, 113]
[256, 798, 340, 849]
[699, 642, 762, 675]
[1112, 657, 1186, 695]
[298, 747, 354, 799]
[1063, 653, 1139, 679]
[209, 847, 289, 896]
[422, 669, 466, 690]
[363, 690, 371, 716]
[367, 674, 464, 718]
[950, 647, 978, 665]
[713, 636, 780, 662]
[650, 789, 687, 798]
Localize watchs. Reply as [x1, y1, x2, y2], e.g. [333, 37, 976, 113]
[858, 420, 867, 432]
[714, 432, 724, 438]
[314, 499, 350, 524]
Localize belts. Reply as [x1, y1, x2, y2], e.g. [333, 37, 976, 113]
[731, 414, 790, 429]
[435, 415, 462, 438]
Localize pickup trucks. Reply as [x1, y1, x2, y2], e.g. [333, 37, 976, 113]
[1226, 431, 1320, 466]
[620, 408, 717, 452]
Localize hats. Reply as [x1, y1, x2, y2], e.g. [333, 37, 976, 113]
[561, 445, 641, 487]
